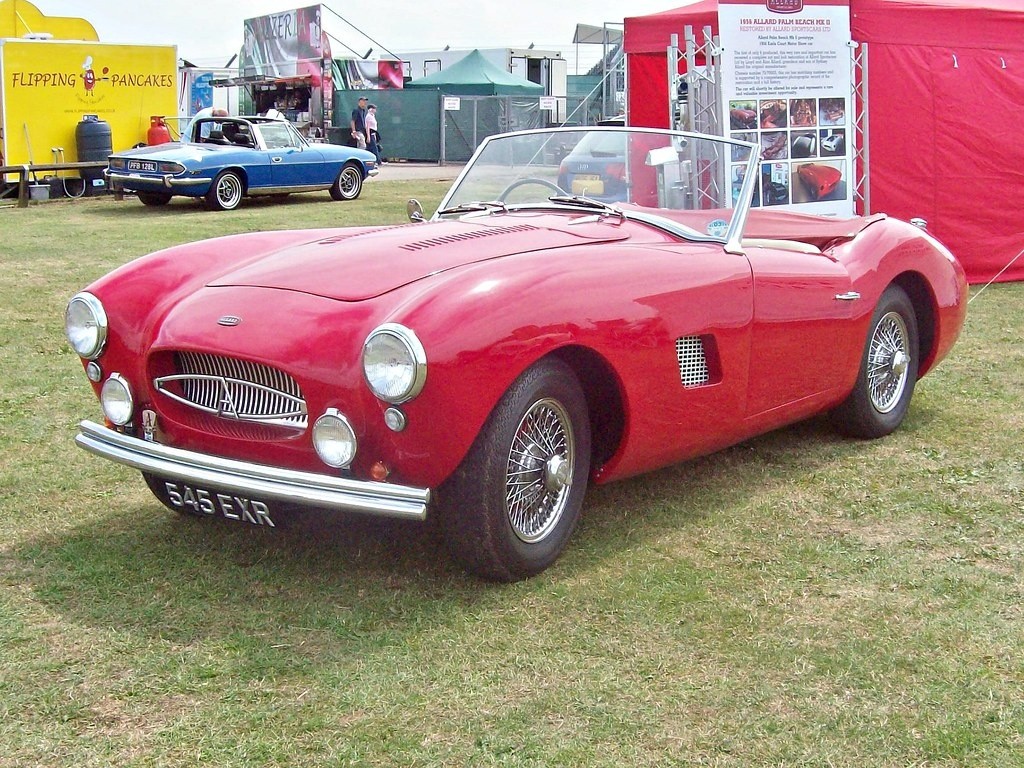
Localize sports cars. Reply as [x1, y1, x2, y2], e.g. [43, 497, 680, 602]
[792, 132, 816, 157]
[796, 162, 842, 202]
[821, 132, 844, 152]
[60, 124, 968, 583]
[102, 115, 380, 212]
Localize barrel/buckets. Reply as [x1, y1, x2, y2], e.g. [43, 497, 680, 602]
[29, 184, 50, 200]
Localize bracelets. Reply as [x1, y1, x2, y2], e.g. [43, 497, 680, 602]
[353, 131, 356, 133]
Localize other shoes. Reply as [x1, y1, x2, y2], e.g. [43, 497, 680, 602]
[379, 161, 388, 166]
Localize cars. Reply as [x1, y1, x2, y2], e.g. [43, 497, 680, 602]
[557, 116, 628, 205]
[730, 104, 756, 128]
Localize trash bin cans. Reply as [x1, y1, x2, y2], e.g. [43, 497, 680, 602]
[324, 126, 356, 148]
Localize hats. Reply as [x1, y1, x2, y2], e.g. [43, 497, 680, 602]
[359, 97, 369, 101]
[367, 104, 378, 109]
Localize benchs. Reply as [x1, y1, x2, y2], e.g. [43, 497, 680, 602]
[0, 160, 124, 207]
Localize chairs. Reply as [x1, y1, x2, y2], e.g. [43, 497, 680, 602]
[230, 134, 255, 147]
[205, 131, 230, 145]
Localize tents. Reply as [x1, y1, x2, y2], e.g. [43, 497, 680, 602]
[403, 49, 545, 95]
[625, 0, 1024, 282]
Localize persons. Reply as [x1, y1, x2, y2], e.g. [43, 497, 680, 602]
[619, 108, 624, 115]
[352, 97, 381, 165]
[181, 108, 227, 202]
